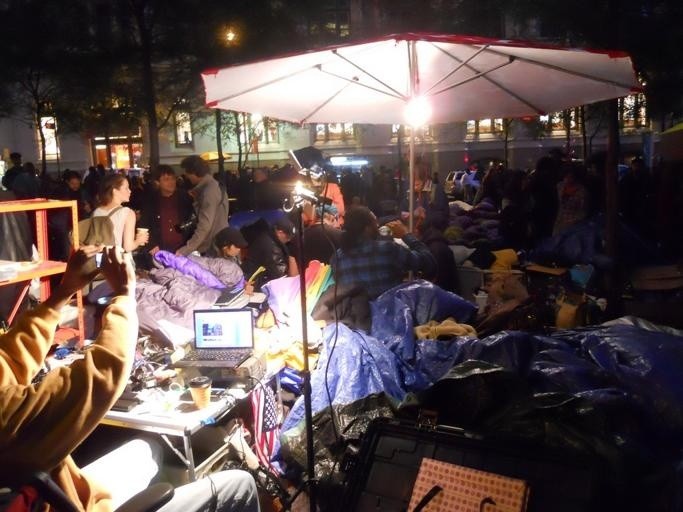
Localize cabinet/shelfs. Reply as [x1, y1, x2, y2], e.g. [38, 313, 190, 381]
[0, 198, 86, 353]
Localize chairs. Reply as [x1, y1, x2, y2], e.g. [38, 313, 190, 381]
[0, 452, 175, 511]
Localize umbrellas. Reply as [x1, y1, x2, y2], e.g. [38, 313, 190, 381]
[200, 26, 646, 281]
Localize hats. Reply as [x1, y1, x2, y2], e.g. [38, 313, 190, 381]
[273, 218, 293, 235]
[215, 227, 248, 250]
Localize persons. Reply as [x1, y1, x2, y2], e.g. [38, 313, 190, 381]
[0, 147, 663, 327]
[0, 242, 261, 512]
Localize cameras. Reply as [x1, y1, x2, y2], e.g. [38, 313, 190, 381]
[95, 250, 133, 270]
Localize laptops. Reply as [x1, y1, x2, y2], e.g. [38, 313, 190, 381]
[169, 308, 255, 369]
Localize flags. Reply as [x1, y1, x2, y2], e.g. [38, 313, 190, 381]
[252, 386, 286, 478]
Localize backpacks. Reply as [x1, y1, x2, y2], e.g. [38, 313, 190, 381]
[67, 207, 122, 246]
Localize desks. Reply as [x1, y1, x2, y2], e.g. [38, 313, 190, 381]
[99, 319, 337, 485]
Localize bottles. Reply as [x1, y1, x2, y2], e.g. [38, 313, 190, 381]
[378, 225, 393, 238]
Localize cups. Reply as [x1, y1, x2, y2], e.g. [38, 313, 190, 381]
[136, 229, 149, 246]
[189, 376, 212, 408]
